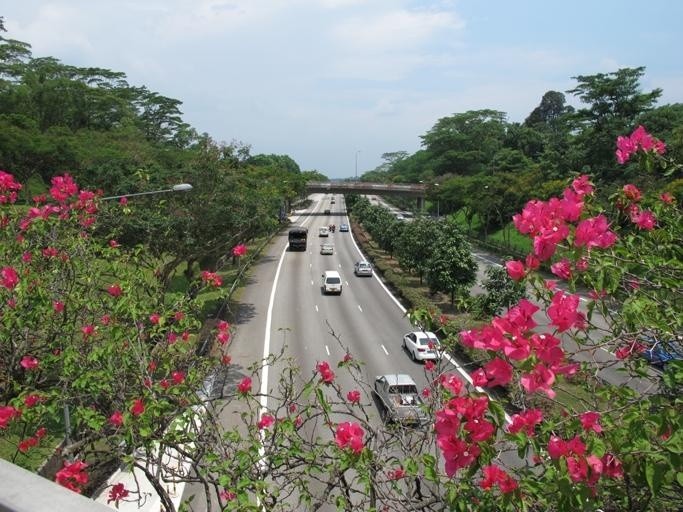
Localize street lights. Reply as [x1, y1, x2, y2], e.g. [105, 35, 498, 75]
[64, 183, 193, 460]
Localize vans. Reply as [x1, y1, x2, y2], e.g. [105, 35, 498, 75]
[322, 271, 342, 294]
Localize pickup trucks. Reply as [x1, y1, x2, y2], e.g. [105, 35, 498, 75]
[375, 374, 427, 429]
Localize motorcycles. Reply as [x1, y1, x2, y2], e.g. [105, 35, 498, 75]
[330, 227, 334, 233]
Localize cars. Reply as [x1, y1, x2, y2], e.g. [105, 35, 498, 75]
[324, 193, 336, 214]
[638, 336, 683, 372]
[320, 244, 333, 255]
[339, 224, 349, 232]
[320, 227, 328, 236]
[354, 261, 372, 276]
[403, 332, 444, 360]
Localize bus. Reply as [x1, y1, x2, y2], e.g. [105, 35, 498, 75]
[289, 228, 308, 251]
